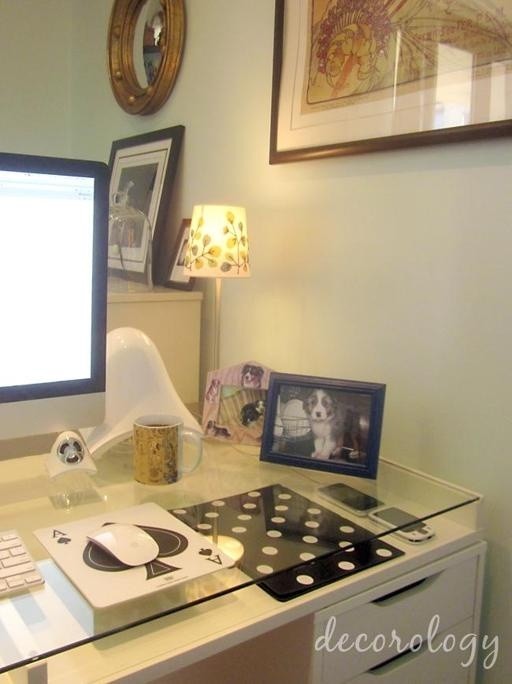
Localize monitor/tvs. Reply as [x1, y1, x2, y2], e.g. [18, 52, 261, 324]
[0, 151, 112, 405]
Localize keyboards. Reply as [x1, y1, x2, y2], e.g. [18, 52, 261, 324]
[1, 533, 45, 597]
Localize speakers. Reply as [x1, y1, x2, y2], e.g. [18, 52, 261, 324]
[44, 429, 98, 478]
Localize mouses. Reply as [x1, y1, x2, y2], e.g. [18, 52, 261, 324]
[86, 522, 161, 567]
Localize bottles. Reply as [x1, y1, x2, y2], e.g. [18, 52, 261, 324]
[106, 182, 156, 296]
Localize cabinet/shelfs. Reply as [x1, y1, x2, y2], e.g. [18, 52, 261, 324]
[0, 441, 489, 683]
[105, 274, 205, 409]
[104, 0, 189, 116]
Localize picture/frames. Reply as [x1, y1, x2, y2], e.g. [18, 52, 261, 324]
[103, 122, 185, 277]
[266, 0, 512, 168]
[162, 217, 198, 289]
[260, 369, 390, 479]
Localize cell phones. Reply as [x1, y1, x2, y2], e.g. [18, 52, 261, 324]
[317, 483, 383, 516]
[369, 506, 436, 543]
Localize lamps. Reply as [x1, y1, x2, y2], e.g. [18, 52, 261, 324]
[180, 196, 250, 434]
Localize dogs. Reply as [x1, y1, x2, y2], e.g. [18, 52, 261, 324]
[241, 364, 264, 389]
[240, 399, 266, 427]
[205, 420, 231, 438]
[303, 387, 370, 460]
[206, 379, 220, 403]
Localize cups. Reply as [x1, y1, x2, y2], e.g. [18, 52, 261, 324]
[132, 414, 202, 488]
